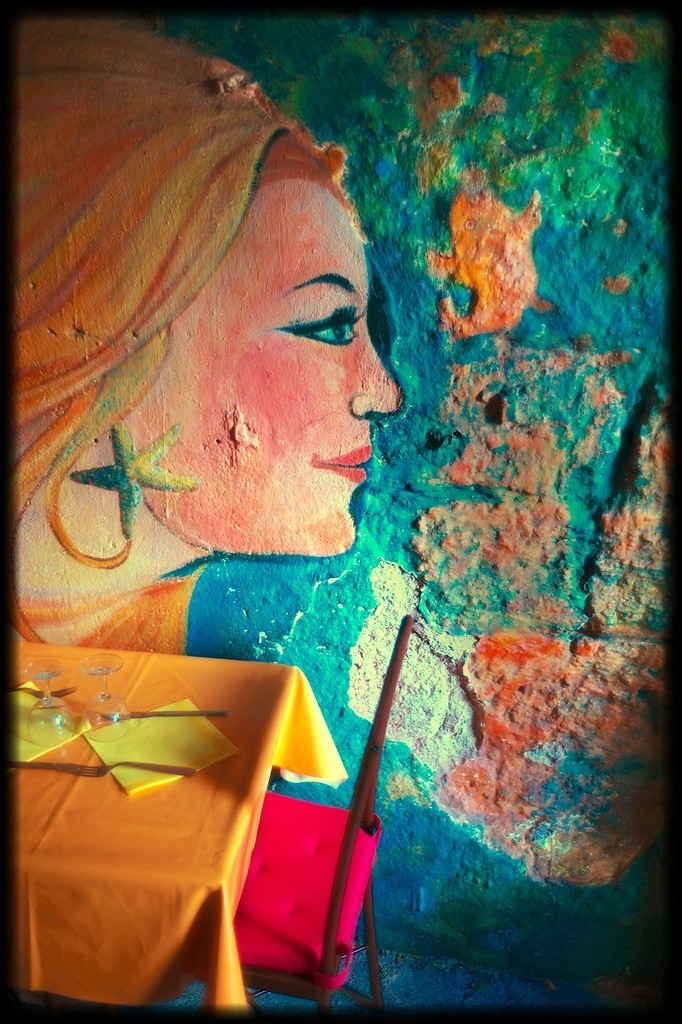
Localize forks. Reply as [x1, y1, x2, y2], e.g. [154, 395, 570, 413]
[68, 762, 196, 781]
[9, 684, 78, 699]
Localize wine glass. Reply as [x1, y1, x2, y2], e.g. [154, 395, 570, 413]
[79, 653, 134, 742]
[23, 659, 75, 749]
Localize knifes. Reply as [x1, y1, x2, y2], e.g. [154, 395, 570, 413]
[7, 760, 78, 772]
[99, 711, 229, 718]
